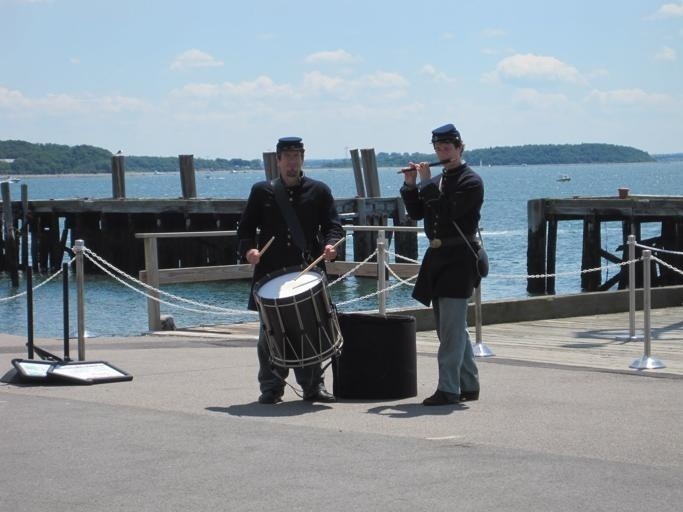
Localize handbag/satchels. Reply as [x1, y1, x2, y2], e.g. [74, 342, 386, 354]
[476, 249, 489, 278]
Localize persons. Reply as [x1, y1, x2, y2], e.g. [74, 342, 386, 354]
[400, 123, 484, 406]
[237, 136, 345, 404]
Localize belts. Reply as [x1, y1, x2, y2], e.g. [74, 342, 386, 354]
[431, 235, 476, 249]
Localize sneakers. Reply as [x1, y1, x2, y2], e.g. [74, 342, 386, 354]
[423, 389, 479, 406]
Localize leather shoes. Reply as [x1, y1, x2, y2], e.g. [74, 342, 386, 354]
[259, 390, 281, 404]
[304, 389, 336, 402]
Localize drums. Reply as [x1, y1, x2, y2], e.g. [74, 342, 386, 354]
[253, 263, 343, 368]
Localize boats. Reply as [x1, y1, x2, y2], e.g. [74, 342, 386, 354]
[147, 168, 256, 182]
[0, 176, 22, 184]
[557, 173, 571, 183]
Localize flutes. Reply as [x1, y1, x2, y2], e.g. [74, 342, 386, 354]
[397, 158, 457, 174]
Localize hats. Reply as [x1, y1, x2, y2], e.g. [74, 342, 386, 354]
[277, 138, 304, 151]
[432, 124, 460, 144]
